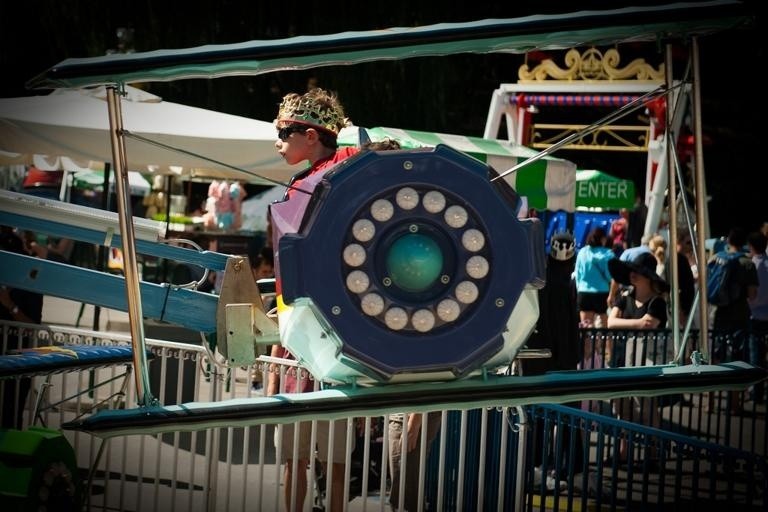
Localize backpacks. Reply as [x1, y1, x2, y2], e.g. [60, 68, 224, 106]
[706, 251, 745, 305]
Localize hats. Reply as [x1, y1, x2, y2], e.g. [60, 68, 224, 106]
[608, 247, 667, 286]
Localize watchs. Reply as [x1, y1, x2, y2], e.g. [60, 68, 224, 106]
[10, 306, 18, 315]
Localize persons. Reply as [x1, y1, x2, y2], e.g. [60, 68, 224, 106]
[521, 194, 767, 479]
[386, 410, 442, 511]
[266, 88, 356, 512]
[162, 246, 275, 296]
[0, 224, 44, 430]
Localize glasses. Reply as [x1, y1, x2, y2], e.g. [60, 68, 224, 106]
[278, 128, 306, 139]
